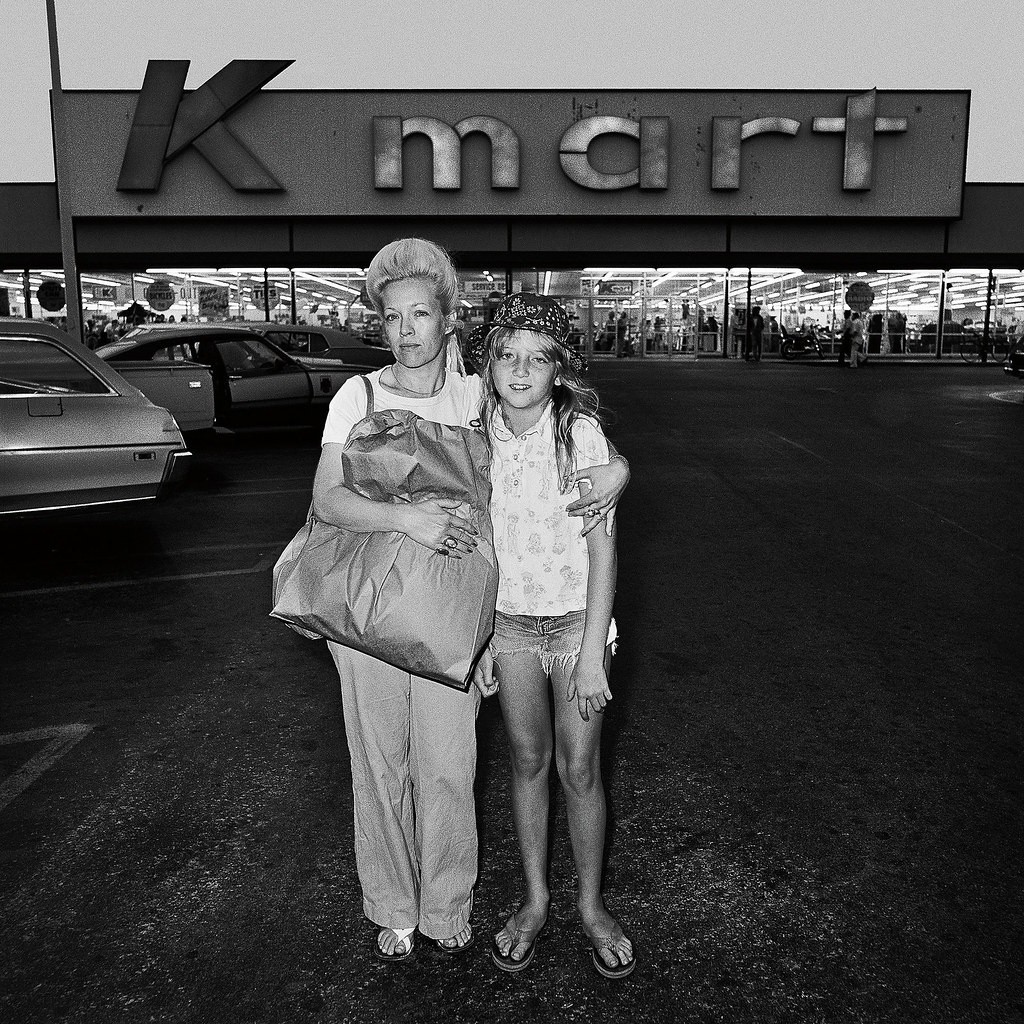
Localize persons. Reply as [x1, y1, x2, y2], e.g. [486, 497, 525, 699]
[632, 316, 666, 354]
[312, 237, 630, 961]
[836, 310, 905, 368]
[596, 311, 628, 357]
[464, 292, 636, 979]
[136, 313, 188, 355]
[47, 316, 67, 332]
[677, 310, 719, 352]
[83, 319, 128, 351]
[731, 306, 778, 364]
[275, 314, 291, 324]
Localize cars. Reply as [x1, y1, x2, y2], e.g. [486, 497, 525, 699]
[1004, 335, 1024, 380]
[0, 316, 194, 518]
[0, 325, 362, 433]
[231, 321, 397, 374]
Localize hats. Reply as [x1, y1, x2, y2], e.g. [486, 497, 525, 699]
[465, 293, 588, 383]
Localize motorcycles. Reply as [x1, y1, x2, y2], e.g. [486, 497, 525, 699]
[778, 322, 827, 360]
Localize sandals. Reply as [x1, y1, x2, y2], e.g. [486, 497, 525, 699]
[372, 923, 417, 963]
[434, 922, 475, 953]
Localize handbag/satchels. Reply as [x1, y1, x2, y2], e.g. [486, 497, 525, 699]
[272, 375, 374, 641]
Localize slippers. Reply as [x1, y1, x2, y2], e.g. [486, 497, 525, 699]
[581, 913, 637, 978]
[490, 910, 549, 972]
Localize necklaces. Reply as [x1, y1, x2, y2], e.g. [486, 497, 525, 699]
[391, 364, 445, 395]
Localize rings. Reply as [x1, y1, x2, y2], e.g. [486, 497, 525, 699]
[436, 547, 449, 556]
[586, 508, 600, 518]
[442, 536, 458, 549]
[595, 513, 606, 520]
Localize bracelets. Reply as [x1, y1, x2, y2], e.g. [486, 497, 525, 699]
[609, 454, 629, 467]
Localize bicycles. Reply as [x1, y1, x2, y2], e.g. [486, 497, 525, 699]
[961, 333, 1009, 363]
